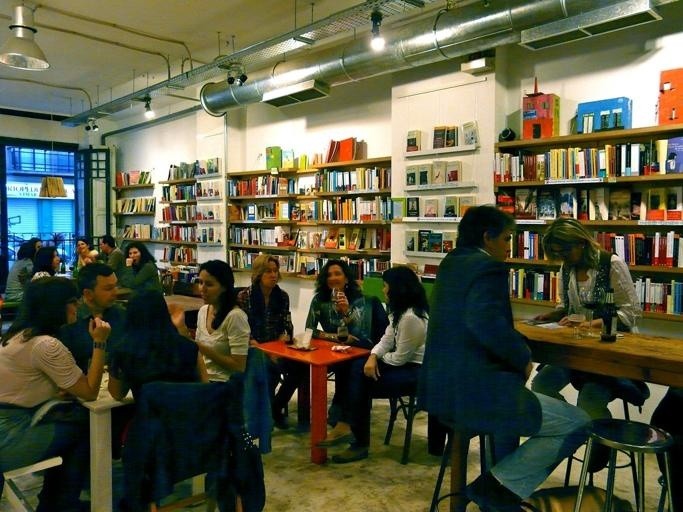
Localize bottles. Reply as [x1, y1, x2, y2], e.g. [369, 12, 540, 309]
[61, 262, 65, 273]
[601, 288, 618, 342]
[72, 263, 78, 277]
[285, 312, 294, 343]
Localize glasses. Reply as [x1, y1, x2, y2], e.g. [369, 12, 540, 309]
[65, 298, 79, 306]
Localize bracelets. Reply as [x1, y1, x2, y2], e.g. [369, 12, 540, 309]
[92, 341, 108, 351]
[344, 309, 353, 317]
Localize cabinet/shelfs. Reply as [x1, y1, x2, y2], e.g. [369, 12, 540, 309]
[228, 156, 391, 282]
[494, 122, 683, 323]
[404, 144, 477, 256]
[110, 173, 196, 265]
[195, 173, 222, 246]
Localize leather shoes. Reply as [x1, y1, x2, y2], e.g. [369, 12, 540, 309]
[464, 470, 525, 511]
[316, 430, 356, 448]
[333, 447, 368, 463]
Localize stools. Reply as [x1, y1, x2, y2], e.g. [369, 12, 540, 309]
[0, 456, 63, 512]
[384, 393, 421, 465]
[430, 381, 675, 512]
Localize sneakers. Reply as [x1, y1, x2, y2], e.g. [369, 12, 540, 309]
[274, 412, 290, 430]
[587, 440, 611, 473]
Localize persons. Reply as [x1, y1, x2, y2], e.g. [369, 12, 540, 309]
[155, 260, 251, 506]
[305, 259, 373, 423]
[57, 262, 129, 375]
[530, 219, 643, 474]
[0, 277, 111, 511]
[4, 235, 164, 317]
[415, 203, 591, 512]
[316, 265, 430, 465]
[232, 253, 299, 427]
[107, 288, 208, 512]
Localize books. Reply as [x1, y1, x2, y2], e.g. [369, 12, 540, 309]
[116, 138, 391, 279]
[405, 119, 481, 281]
[492, 138, 683, 317]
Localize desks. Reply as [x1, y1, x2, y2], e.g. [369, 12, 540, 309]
[256, 338, 370, 464]
[79, 372, 134, 512]
[511, 319, 683, 387]
[164, 294, 206, 312]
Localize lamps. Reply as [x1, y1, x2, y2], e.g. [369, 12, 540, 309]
[371, 12, 386, 51]
[38, 95, 68, 198]
[144, 93, 154, 119]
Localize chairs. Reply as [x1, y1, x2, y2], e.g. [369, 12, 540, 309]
[140, 381, 244, 512]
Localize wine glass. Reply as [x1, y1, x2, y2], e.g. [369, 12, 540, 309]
[338, 326, 348, 346]
[579, 287, 604, 339]
[330, 287, 341, 307]
[567, 289, 585, 339]
